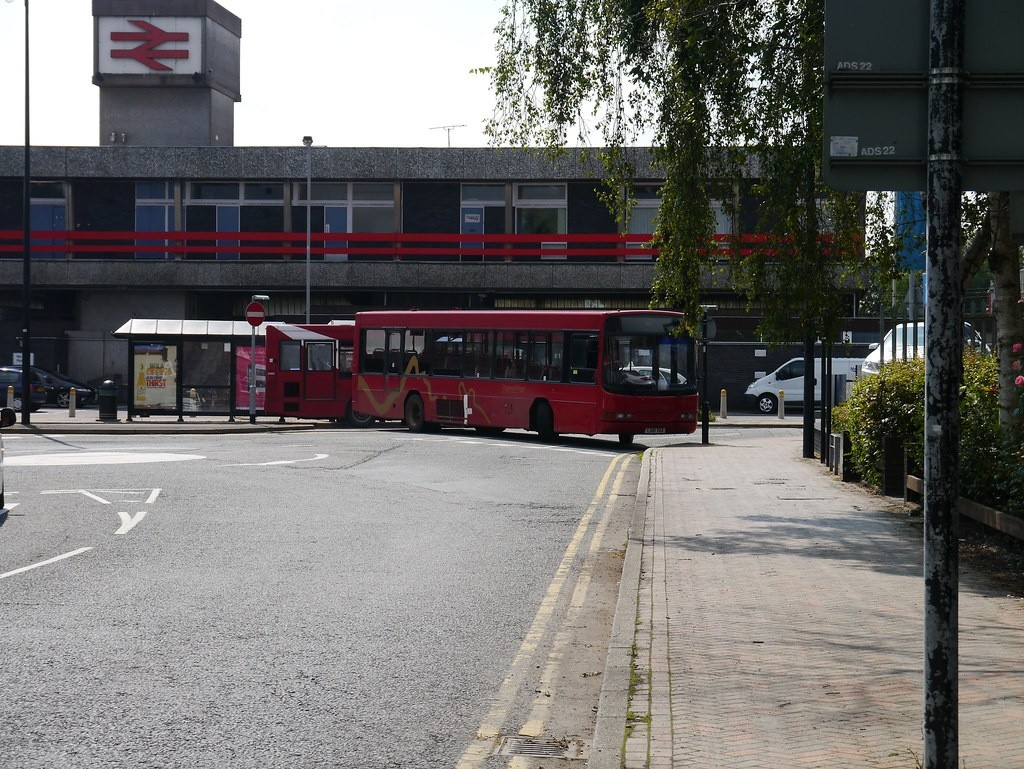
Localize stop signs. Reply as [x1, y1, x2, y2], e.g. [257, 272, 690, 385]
[246, 302, 266, 327]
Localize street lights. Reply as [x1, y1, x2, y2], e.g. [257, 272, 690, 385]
[303, 135, 314, 325]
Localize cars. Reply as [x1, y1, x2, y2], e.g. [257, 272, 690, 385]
[861, 322, 992, 386]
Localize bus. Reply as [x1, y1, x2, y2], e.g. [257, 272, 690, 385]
[264, 326, 377, 427]
[353, 309, 699, 451]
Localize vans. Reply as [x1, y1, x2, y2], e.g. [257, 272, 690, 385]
[2, 366, 98, 408]
[743, 358, 865, 414]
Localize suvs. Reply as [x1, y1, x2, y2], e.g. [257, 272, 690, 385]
[0, 366, 47, 411]
